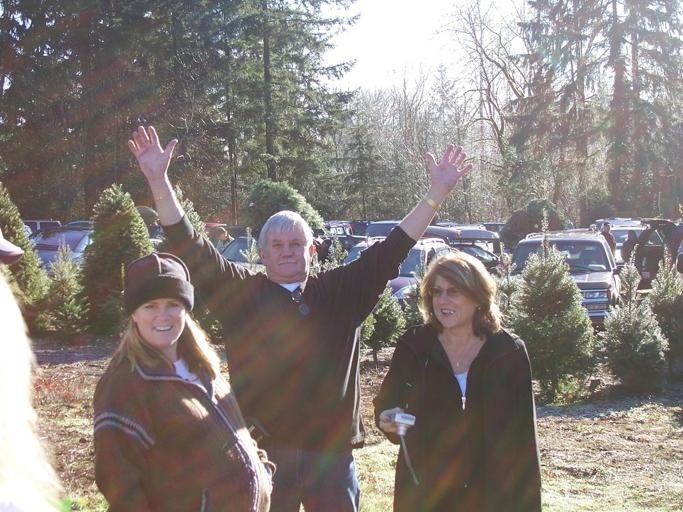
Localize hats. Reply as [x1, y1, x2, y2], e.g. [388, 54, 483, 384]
[124, 254, 195, 315]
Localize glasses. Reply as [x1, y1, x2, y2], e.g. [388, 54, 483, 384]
[429, 286, 462, 299]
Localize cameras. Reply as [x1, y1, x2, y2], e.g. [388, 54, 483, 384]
[390, 413, 416, 437]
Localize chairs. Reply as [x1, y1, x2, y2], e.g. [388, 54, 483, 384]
[578, 249, 599, 264]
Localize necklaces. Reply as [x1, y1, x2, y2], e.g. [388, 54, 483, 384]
[443, 330, 473, 368]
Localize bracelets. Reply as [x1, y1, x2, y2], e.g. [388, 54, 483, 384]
[422, 194, 440, 211]
[153, 189, 175, 202]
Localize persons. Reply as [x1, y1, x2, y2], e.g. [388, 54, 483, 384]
[91, 252, 276, 512]
[0, 227, 66, 512]
[599, 222, 616, 260]
[214, 226, 235, 254]
[371, 253, 541, 512]
[126, 123, 473, 512]
[621, 230, 639, 264]
